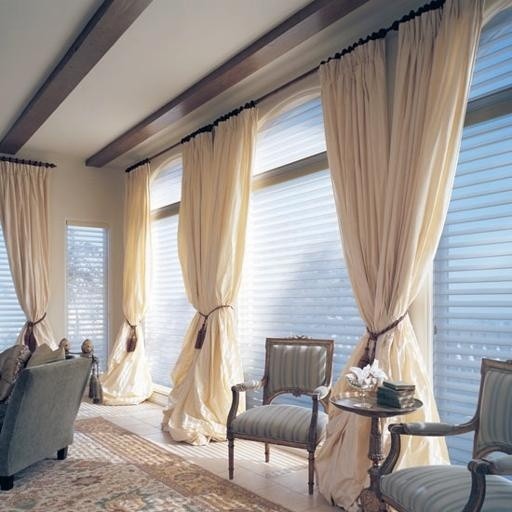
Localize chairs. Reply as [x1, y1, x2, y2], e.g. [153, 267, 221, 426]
[368, 356, 512, 512]
[226, 336, 333, 496]
[0, 356, 94, 491]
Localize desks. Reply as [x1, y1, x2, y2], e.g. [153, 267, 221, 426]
[330, 391, 421, 512]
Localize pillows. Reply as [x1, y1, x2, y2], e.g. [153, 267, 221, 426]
[0, 343, 31, 402]
[25, 343, 65, 370]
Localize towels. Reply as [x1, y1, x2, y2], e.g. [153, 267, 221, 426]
[346, 359, 387, 391]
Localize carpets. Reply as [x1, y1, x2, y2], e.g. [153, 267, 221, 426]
[0, 400, 291, 512]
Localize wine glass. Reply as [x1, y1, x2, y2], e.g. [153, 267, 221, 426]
[349, 379, 378, 408]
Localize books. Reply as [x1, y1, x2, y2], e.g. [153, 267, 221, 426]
[374, 379, 416, 409]
